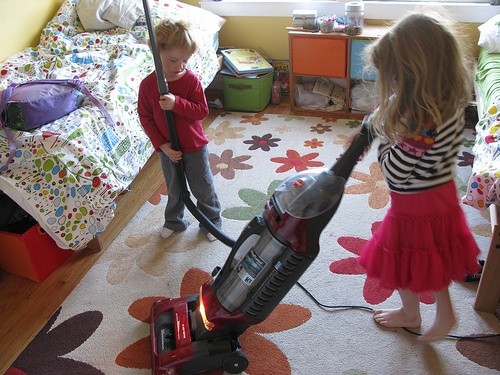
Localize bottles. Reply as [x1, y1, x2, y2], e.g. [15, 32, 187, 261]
[344, 2, 364, 35]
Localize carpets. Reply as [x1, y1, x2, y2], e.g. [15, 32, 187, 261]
[0, 111, 500, 375]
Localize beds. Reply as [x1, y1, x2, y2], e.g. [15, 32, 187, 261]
[0, 0, 232, 251]
[461, 13, 500, 312]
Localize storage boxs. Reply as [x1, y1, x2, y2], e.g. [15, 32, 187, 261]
[0, 223, 77, 282]
[222, 70, 273, 112]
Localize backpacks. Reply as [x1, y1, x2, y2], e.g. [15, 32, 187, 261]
[0, 79, 85, 131]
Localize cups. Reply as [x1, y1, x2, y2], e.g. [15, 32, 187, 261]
[318, 18, 335, 33]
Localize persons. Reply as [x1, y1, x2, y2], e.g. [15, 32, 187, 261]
[138, 19, 222, 242]
[342, 14, 481, 344]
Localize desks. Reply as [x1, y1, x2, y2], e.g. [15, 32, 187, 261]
[287, 25, 391, 114]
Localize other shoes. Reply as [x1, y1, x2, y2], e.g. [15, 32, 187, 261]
[464, 258, 484, 281]
